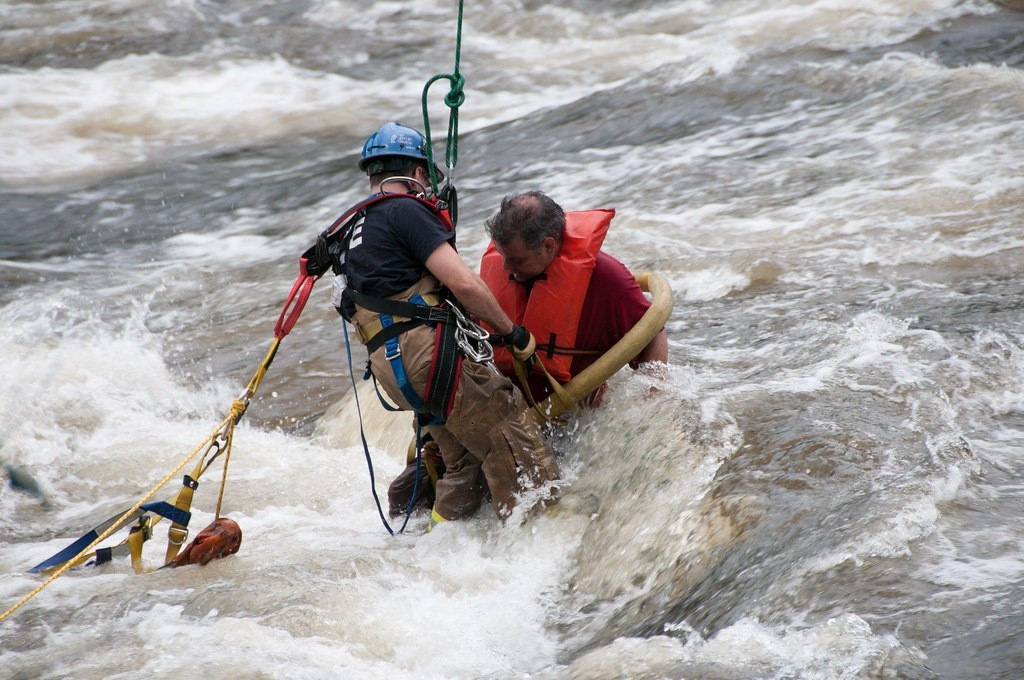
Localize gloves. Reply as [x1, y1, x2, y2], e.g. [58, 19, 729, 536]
[501, 322, 537, 369]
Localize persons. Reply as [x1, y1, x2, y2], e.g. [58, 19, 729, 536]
[331, 122, 562, 533]
[388, 191, 669, 518]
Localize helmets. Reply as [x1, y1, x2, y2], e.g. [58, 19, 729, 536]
[358, 121, 444, 186]
